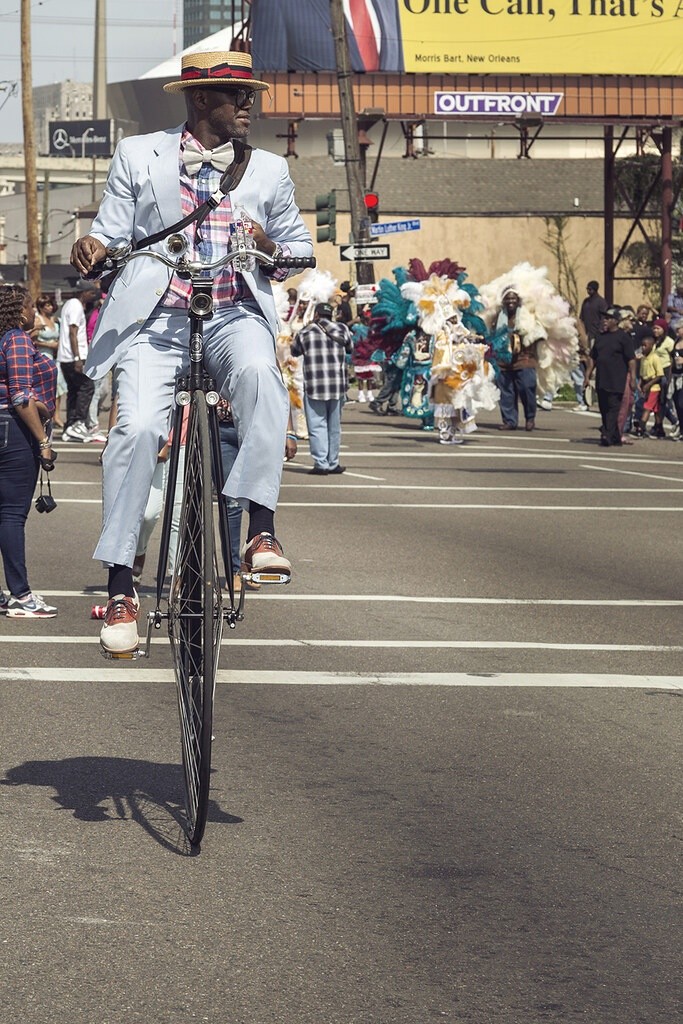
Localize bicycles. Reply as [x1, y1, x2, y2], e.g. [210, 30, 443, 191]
[83, 238, 316, 846]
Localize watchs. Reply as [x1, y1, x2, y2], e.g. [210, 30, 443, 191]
[74, 356, 81, 362]
[271, 241, 283, 259]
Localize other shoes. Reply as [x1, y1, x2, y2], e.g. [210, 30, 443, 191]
[345, 393, 402, 416]
[329, 465, 346, 473]
[499, 422, 517, 430]
[526, 419, 535, 430]
[308, 468, 329, 475]
[598, 423, 682, 446]
[226, 575, 261, 592]
[573, 404, 588, 411]
[132, 572, 142, 585]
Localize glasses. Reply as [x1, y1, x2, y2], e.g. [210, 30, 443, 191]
[200, 88, 256, 108]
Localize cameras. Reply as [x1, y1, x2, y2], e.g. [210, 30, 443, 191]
[35, 495, 57, 513]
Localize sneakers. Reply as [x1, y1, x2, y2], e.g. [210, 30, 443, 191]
[6, 593, 57, 618]
[66, 420, 92, 440]
[62, 433, 81, 442]
[0, 589, 10, 613]
[240, 532, 291, 575]
[101, 587, 140, 653]
[83, 426, 107, 442]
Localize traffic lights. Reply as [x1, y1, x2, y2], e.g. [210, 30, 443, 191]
[314, 190, 337, 242]
[363, 190, 380, 240]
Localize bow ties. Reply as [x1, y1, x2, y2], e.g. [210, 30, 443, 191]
[183, 141, 234, 176]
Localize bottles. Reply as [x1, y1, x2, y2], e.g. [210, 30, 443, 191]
[228, 202, 255, 272]
[90, 606, 107, 618]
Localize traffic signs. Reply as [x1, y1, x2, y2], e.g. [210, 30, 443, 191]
[339, 243, 391, 260]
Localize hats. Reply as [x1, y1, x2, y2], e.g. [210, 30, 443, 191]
[341, 281, 356, 292]
[603, 309, 620, 320]
[316, 303, 333, 316]
[162, 51, 270, 96]
[653, 319, 668, 332]
[72, 279, 93, 292]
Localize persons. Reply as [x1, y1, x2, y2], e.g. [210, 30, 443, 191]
[0, 258, 683, 618]
[250, 0, 404, 73]
[70, 51, 315, 654]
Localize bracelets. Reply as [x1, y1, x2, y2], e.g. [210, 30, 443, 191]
[39, 442, 52, 449]
[39, 436, 50, 444]
